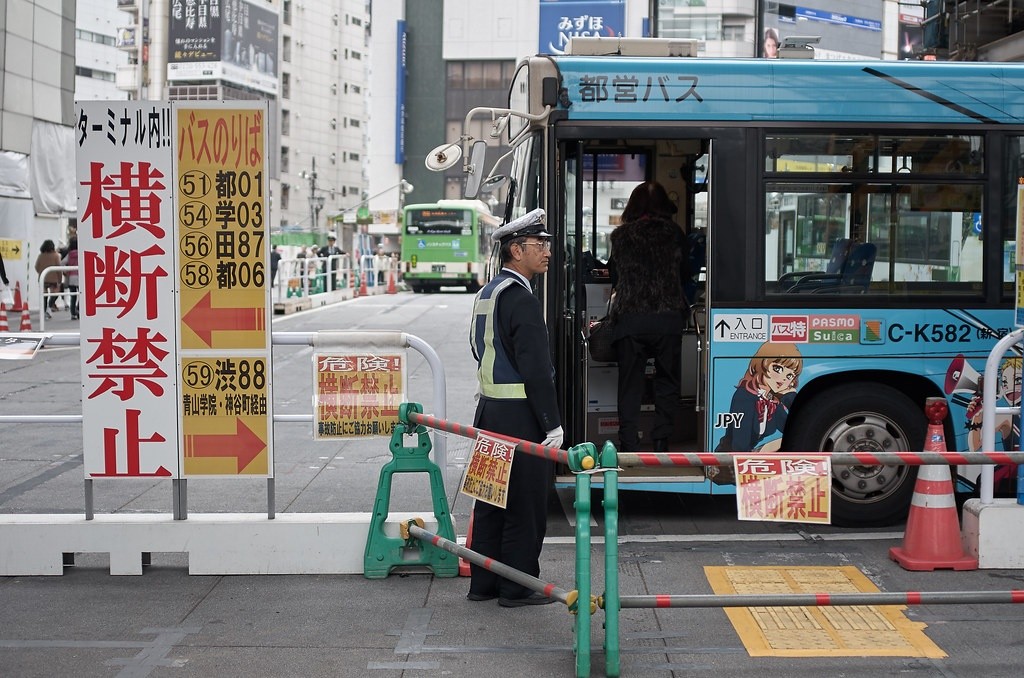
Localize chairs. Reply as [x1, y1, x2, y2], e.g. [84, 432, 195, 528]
[777, 237, 878, 296]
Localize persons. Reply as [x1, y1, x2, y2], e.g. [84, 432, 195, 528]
[762, 28, 779, 58]
[271, 237, 401, 293]
[607, 180, 696, 452]
[35, 224, 79, 321]
[467, 208, 564, 608]
[0, 253, 10, 286]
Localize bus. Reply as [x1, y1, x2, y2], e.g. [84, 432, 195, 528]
[426, 33, 1024, 528]
[776, 192, 983, 288]
[400, 200, 505, 293]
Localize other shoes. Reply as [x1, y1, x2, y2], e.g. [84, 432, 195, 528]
[48, 304, 59, 312]
[45, 312, 51, 319]
[71, 315, 79, 320]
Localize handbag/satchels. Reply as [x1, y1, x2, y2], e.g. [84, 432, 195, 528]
[589, 314, 616, 363]
[0, 286, 15, 305]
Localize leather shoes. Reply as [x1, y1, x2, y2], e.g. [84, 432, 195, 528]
[467, 589, 500, 601]
[498, 591, 557, 608]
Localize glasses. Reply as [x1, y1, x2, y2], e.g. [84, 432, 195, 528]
[522, 241, 551, 251]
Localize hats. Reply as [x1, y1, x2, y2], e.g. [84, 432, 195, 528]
[491, 208, 553, 238]
[328, 236, 336, 241]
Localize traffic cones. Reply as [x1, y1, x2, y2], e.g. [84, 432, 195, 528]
[0, 304, 10, 333]
[358, 272, 368, 294]
[10, 282, 23, 312]
[889, 397, 977, 570]
[19, 302, 32, 332]
[387, 273, 396, 293]
[458, 496, 476, 575]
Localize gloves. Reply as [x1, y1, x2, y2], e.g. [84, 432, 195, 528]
[541, 425, 563, 448]
